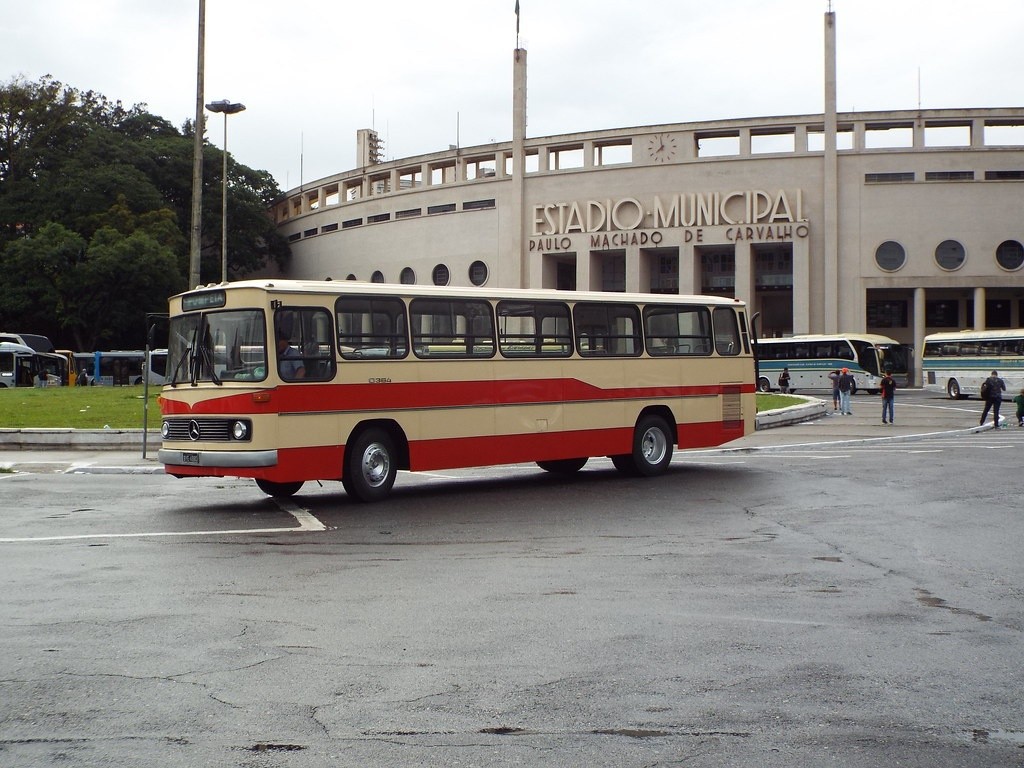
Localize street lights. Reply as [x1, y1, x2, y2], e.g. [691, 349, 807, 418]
[205, 99, 246, 282]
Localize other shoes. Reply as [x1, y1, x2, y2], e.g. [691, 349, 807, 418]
[847, 412, 852, 415]
[890, 420, 893, 423]
[882, 419, 887, 423]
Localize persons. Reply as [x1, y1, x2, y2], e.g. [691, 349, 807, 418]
[779, 368, 790, 393]
[277, 331, 306, 379]
[827, 370, 841, 410]
[1012, 389, 1024, 426]
[979, 371, 1006, 427]
[78, 369, 88, 386]
[90, 378, 95, 386]
[837, 368, 853, 415]
[880, 369, 896, 423]
[37, 367, 50, 387]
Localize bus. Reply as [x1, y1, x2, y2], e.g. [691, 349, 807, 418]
[23, 351, 78, 385]
[146, 278, 760, 503]
[0, 333, 69, 389]
[728, 333, 913, 394]
[358, 344, 607, 355]
[921, 329, 1024, 400]
[73, 351, 146, 386]
[147, 344, 364, 387]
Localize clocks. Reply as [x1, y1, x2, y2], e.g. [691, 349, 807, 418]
[648, 132, 677, 163]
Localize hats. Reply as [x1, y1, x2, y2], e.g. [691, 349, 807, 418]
[842, 368, 848, 373]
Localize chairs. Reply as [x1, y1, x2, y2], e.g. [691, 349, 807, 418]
[777, 352, 853, 359]
[580, 343, 709, 356]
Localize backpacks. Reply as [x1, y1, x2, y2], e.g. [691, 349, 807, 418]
[981, 378, 994, 401]
[884, 379, 894, 398]
